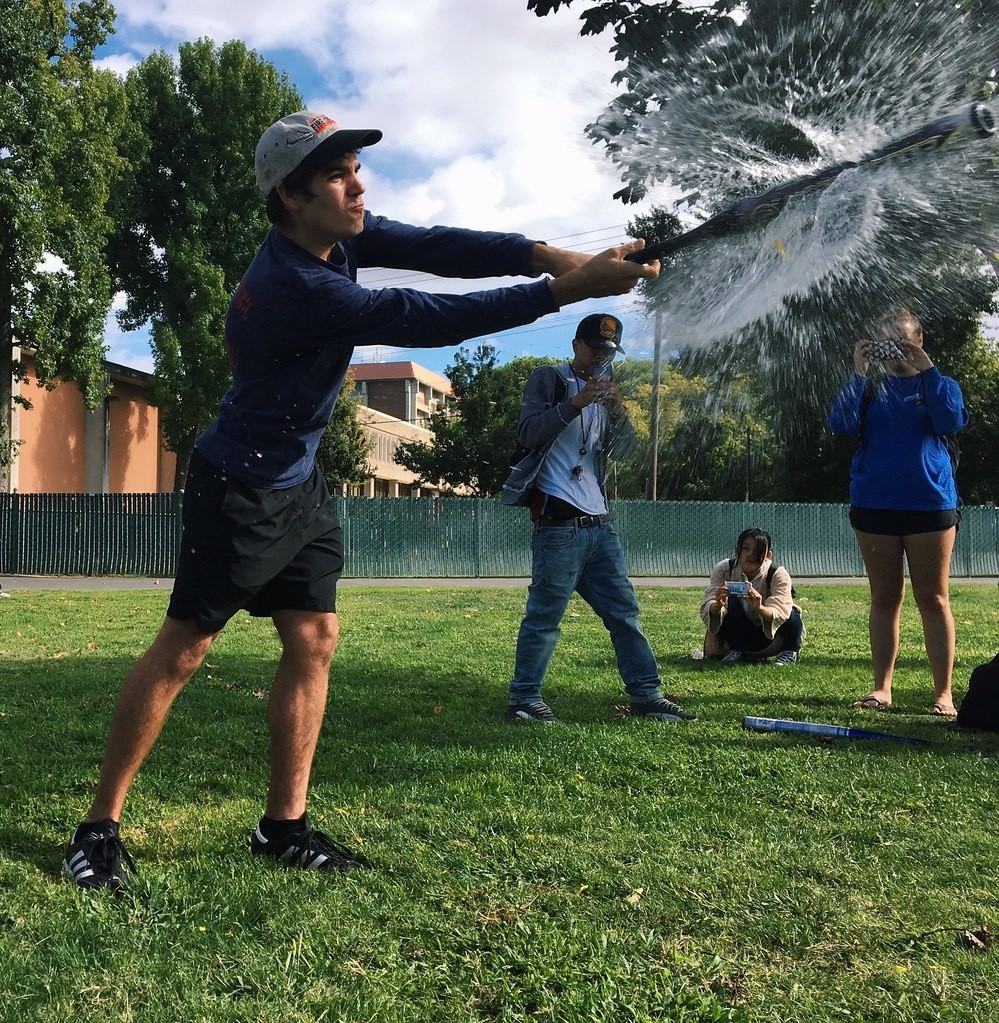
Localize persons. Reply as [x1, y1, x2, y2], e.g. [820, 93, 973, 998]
[56, 110, 662, 900]
[826, 306, 970, 717]
[501, 312, 699, 725]
[700, 527, 808, 668]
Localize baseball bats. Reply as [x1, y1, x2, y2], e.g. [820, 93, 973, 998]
[623, 101, 995, 264]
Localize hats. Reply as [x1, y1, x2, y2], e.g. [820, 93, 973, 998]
[577, 311, 627, 355]
[254, 112, 383, 195]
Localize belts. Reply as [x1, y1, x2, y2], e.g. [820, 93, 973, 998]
[534, 514, 617, 527]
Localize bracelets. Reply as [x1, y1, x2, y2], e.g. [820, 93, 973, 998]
[611, 406, 629, 420]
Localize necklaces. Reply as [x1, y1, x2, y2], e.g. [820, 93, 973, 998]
[568, 363, 597, 482]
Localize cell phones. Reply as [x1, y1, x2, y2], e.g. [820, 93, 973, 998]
[595, 361, 614, 403]
[863, 338, 906, 360]
[725, 580, 750, 595]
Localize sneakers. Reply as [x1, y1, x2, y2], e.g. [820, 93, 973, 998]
[61, 819, 136, 900]
[631, 698, 699, 724]
[772, 651, 799, 667]
[720, 650, 746, 664]
[510, 700, 563, 726]
[251, 820, 369, 874]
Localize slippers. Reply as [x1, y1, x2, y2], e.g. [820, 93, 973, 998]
[931, 704, 958, 718]
[850, 697, 893, 711]
